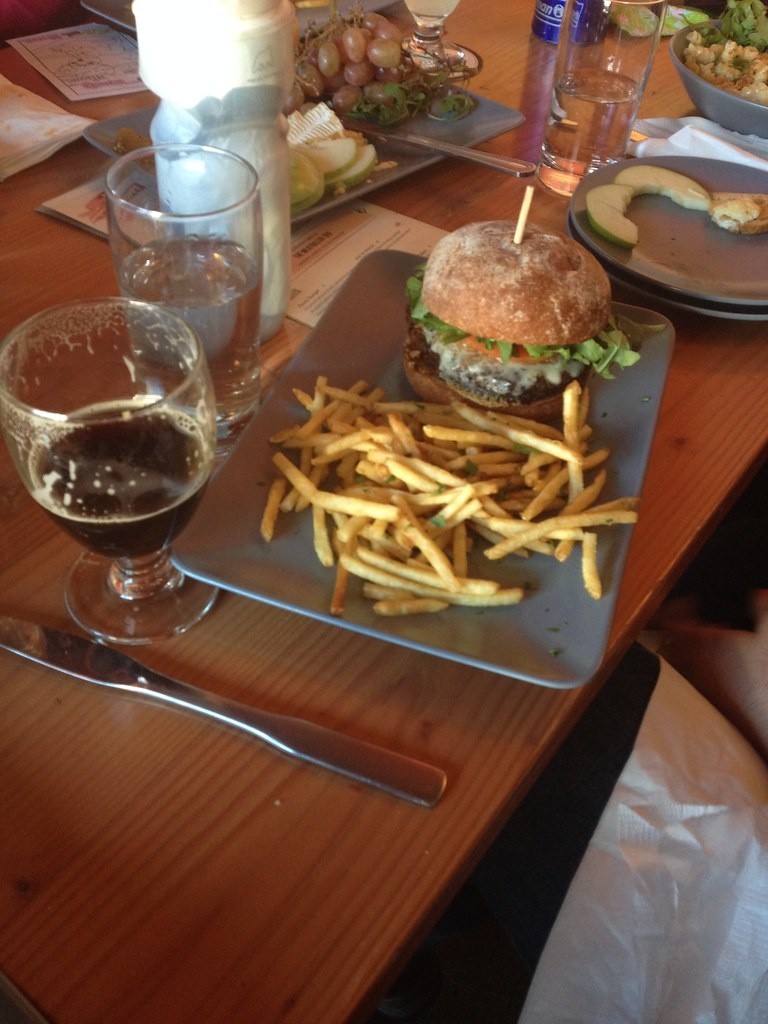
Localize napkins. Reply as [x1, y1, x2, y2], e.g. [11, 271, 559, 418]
[0, 73, 99, 186]
[626, 116, 767, 171]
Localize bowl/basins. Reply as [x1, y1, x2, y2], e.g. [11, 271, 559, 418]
[668, 18, 768, 140]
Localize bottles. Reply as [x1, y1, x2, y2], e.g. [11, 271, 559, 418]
[126, 0, 299, 348]
[514, 1, 608, 171]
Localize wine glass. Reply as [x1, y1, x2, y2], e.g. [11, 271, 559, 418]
[401, 0, 483, 80]
[0, 298, 220, 645]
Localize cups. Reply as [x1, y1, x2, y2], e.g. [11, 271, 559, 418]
[535, 0, 670, 196]
[104, 141, 265, 440]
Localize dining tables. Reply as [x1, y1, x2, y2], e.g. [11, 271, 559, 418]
[0, 0, 767, 1024]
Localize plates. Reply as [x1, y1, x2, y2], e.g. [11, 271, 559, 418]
[165, 249, 675, 686]
[82, 78, 529, 226]
[80, 0, 405, 42]
[564, 155, 768, 324]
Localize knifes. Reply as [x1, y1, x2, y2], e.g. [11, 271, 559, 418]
[0, 616, 449, 807]
